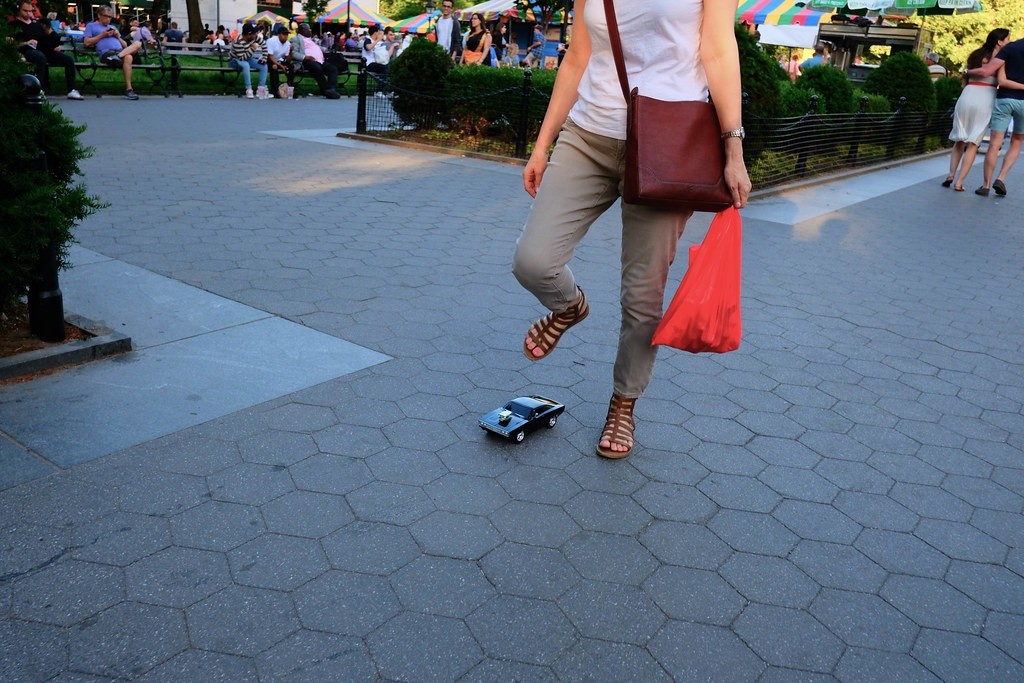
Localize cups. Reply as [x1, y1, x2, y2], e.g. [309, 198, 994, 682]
[286, 87, 294, 99]
[258, 86, 265, 99]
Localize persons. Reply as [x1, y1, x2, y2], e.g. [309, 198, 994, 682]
[435, 0, 521, 67]
[200, 24, 238, 51]
[161, 21, 188, 51]
[312, 29, 359, 51]
[512, 0, 753, 460]
[121, 19, 156, 47]
[267, 29, 299, 99]
[228, 24, 274, 100]
[925, 52, 947, 85]
[522, 23, 546, 68]
[361, 25, 414, 99]
[84, 5, 142, 100]
[777, 43, 831, 81]
[6, 1, 83, 100]
[941, 28, 1024, 191]
[962, 36, 1024, 196]
[289, 22, 339, 99]
[555, 26, 572, 66]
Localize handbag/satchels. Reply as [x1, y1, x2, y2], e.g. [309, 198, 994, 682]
[623, 86, 734, 213]
[463, 49, 482, 65]
[324, 52, 349, 72]
[651, 201, 742, 354]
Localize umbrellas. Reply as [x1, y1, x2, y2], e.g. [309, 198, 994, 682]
[806, 0, 984, 55]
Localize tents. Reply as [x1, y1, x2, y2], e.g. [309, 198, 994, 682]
[379, 8, 463, 33]
[293, 0, 394, 26]
[456, 0, 574, 24]
[235, 11, 290, 30]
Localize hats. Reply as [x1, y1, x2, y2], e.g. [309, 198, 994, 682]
[533, 25, 543, 30]
[242, 23, 259, 35]
[277, 27, 291, 34]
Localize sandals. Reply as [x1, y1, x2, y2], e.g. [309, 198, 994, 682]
[954, 183, 965, 191]
[596, 392, 638, 459]
[523, 284, 590, 361]
[942, 176, 954, 188]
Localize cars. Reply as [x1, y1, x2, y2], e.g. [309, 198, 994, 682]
[477, 392, 565, 444]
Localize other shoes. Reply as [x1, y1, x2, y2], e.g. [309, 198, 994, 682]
[124, 89, 139, 100]
[246, 89, 254, 99]
[992, 179, 1006, 195]
[320, 88, 340, 99]
[975, 186, 990, 196]
[270, 91, 282, 99]
[105, 54, 123, 69]
[255, 89, 274, 99]
[376, 90, 399, 101]
[67, 89, 84, 100]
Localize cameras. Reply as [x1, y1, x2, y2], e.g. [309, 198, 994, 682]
[108, 27, 114, 31]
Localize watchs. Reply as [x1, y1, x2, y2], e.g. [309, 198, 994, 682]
[963, 69, 968, 75]
[720, 126, 746, 140]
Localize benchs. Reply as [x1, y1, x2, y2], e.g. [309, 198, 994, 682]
[17, 35, 390, 99]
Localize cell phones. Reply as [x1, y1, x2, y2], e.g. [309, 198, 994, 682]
[258, 60, 265, 65]
